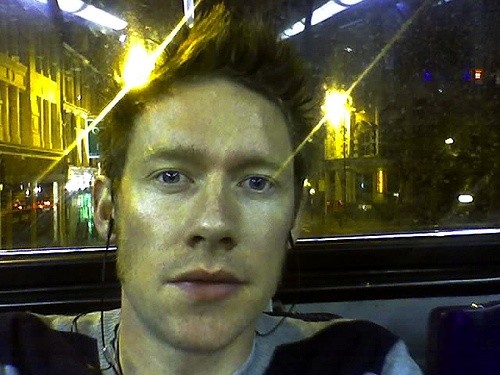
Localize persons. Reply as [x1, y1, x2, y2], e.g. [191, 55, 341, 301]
[0, 4, 425, 375]
